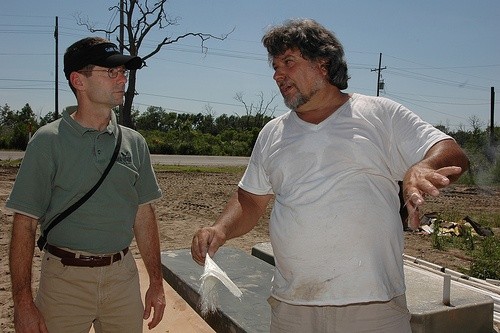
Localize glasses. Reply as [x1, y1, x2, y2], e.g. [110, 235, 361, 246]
[78, 67, 128, 79]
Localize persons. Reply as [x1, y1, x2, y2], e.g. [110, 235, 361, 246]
[6, 37, 166, 333]
[191, 17, 475, 333]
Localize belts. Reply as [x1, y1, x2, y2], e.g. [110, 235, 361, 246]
[45, 243, 129, 268]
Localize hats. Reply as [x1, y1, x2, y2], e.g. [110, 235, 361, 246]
[64, 43, 142, 80]
